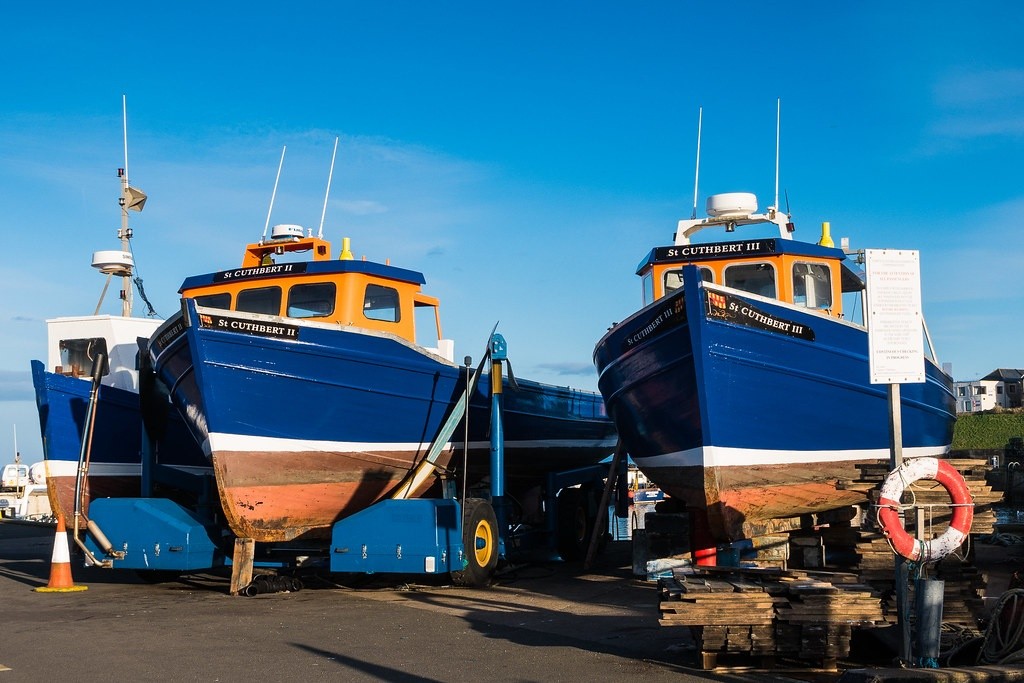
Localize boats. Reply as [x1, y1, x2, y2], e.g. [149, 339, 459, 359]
[590, 93, 958, 521]
[144, 135, 643, 547]
[0, 425, 60, 525]
[27, 95, 196, 532]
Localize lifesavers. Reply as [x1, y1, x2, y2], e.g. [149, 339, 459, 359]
[873, 456, 976, 564]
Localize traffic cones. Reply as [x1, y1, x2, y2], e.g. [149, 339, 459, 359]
[37, 511, 91, 595]
